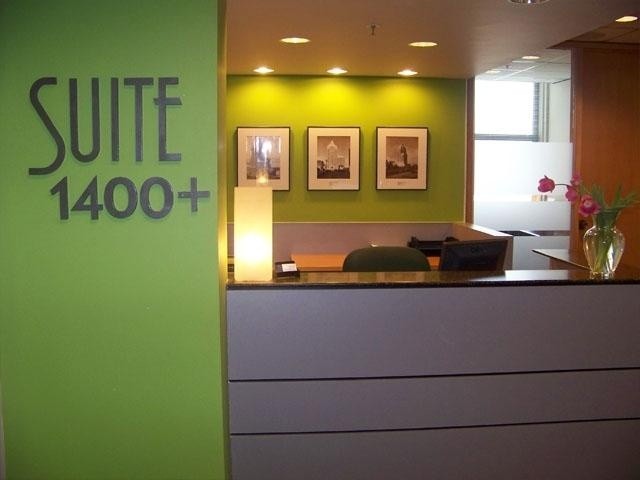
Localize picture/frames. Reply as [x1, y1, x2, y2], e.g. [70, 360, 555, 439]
[235, 124, 292, 193]
[306, 126, 362, 192]
[376, 126, 429, 191]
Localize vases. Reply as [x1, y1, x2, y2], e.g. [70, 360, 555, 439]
[582, 210, 626, 277]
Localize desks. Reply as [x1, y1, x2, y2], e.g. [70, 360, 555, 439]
[291, 251, 442, 272]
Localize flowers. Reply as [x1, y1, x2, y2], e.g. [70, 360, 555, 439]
[537, 174, 637, 274]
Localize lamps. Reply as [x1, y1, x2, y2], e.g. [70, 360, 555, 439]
[232, 187, 274, 282]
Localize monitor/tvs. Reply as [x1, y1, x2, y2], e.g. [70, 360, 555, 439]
[436, 237, 509, 273]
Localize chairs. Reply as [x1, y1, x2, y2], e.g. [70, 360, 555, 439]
[341, 247, 431, 271]
[438, 238, 508, 271]
